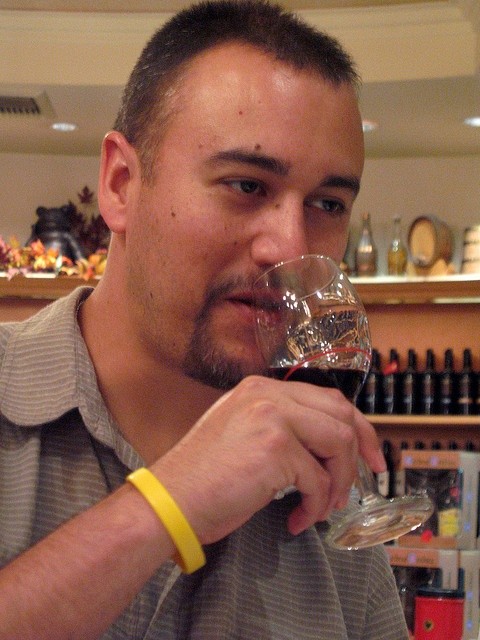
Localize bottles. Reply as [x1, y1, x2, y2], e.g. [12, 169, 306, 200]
[376, 439, 394, 499]
[383, 348, 403, 413]
[439, 349, 459, 415]
[404, 347, 422, 414]
[387, 215, 407, 275]
[476, 371, 480, 414]
[397, 438, 480, 535]
[459, 347, 475, 414]
[362, 348, 383, 413]
[422, 348, 438, 414]
[355, 212, 377, 276]
[396, 566, 416, 629]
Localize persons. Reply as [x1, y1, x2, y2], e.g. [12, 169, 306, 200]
[0, 0, 413, 636]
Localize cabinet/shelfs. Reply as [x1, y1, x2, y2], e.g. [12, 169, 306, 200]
[339, 272, 480, 640]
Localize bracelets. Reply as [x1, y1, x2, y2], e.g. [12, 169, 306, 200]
[124, 465, 208, 576]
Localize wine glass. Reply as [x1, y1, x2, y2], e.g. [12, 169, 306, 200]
[251, 253, 437, 552]
